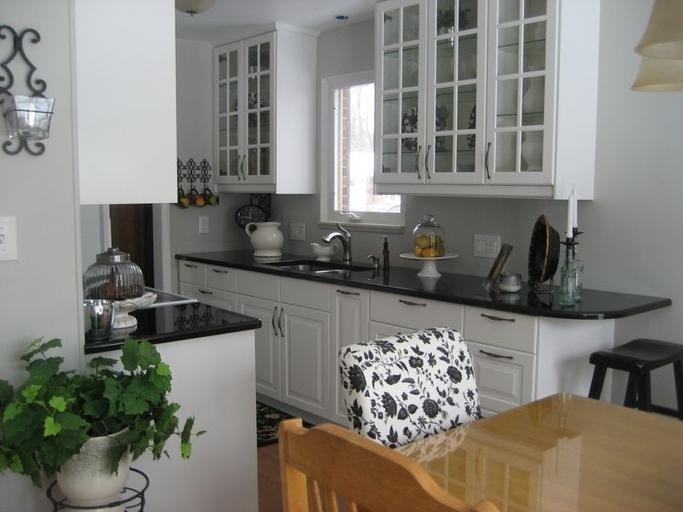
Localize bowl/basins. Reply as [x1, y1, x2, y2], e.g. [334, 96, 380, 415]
[527, 214, 561, 288]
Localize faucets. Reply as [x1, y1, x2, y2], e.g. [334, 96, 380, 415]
[367, 254, 381, 268]
[321, 221, 353, 263]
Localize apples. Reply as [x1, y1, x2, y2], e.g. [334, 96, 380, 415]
[414, 236, 444, 257]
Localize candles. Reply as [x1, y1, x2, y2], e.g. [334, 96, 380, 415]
[566, 190, 574, 239]
[571, 184, 578, 228]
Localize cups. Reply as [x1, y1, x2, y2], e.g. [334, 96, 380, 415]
[494, 293, 521, 307]
[497, 272, 522, 293]
[310, 244, 338, 261]
[82, 297, 112, 341]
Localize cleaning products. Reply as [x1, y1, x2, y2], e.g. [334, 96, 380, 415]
[382, 235, 390, 271]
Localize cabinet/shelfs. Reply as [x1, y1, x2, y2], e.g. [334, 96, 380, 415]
[456, 303, 616, 420]
[369, 1, 486, 201]
[232, 265, 336, 431]
[480, 0, 604, 202]
[362, 285, 464, 351]
[326, 278, 370, 425]
[205, 18, 320, 199]
[175, 256, 238, 315]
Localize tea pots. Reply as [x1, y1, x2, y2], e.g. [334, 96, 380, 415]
[244, 221, 283, 257]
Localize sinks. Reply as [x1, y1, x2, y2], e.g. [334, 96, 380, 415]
[317, 270, 356, 275]
[262, 259, 333, 271]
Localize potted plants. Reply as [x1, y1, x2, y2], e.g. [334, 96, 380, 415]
[0, 329, 206, 512]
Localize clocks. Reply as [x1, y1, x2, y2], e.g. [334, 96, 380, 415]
[234, 204, 268, 233]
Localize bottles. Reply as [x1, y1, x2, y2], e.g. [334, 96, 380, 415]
[523, 66, 543, 126]
[521, 132, 542, 171]
[410, 215, 447, 258]
[572, 236, 583, 303]
[559, 245, 575, 306]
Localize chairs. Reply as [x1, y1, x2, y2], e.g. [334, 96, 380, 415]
[272, 413, 508, 508]
[333, 325, 486, 458]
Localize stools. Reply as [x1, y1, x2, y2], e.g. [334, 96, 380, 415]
[582, 335, 682, 424]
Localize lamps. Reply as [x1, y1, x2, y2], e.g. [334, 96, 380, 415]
[1, 25, 58, 156]
[174, 0, 217, 18]
[629, 2, 681, 96]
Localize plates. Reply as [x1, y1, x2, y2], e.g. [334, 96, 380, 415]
[401, 106, 449, 154]
[465, 105, 475, 148]
[233, 93, 264, 128]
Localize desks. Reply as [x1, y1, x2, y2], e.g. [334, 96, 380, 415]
[350, 386, 682, 512]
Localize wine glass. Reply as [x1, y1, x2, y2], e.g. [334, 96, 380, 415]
[110, 290, 159, 330]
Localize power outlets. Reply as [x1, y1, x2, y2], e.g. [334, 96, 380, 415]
[0, 215, 19, 263]
